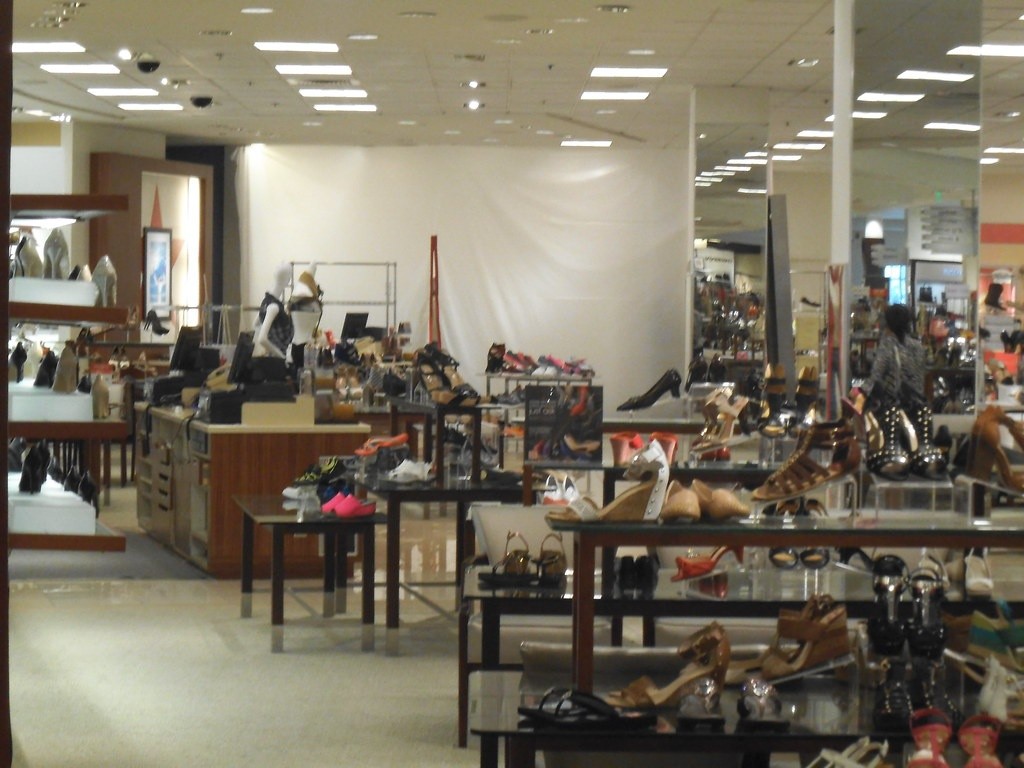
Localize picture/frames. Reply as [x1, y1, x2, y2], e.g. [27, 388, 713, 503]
[143, 227, 173, 322]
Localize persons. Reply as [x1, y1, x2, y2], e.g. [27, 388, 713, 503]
[288, 262, 323, 366]
[253, 263, 295, 364]
[866, 305, 926, 403]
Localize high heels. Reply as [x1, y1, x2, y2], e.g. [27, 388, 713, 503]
[10, 228, 119, 308]
[127, 304, 138, 327]
[8, 326, 158, 420]
[615, 554, 661, 602]
[868, 553, 947, 657]
[404, 336, 482, 410]
[835, 544, 906, 576]
[942, 545, 996, 610]
[721, 592, 851, 691]
[492, 531, 568, 582]
[488, 340, 605, 458]
[143, 309, 170, 335]
[670, 544, 746, 583]
[603, 622, 730, 711]
[541, 352, 1023, 529]
[8, 436, 99, 518]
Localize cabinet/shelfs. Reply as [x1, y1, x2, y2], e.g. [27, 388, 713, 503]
[1, 194, 134, 552]
[457, 512, 1024, 768]
[458, 410, 1024, 665]
[483, 372, 591, 471]
[318, 395, 555, 627]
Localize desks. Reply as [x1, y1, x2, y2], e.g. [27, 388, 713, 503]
[134, 402, 369, 579]
[229, 493, 387, 655]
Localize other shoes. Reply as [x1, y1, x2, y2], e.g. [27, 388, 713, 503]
[969, 597, 1024, 674]
[801, 296, 822, 307]
[331, 363, 403, 397]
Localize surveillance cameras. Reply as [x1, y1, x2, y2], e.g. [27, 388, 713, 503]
[191, 95, 212, 107]
[136, 54, 160, 72]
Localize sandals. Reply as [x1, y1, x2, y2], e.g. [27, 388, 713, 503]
[865, 656, 965, 733]
[283, 426, 504, 516]
[769, 495, 830, 570]
[908, 706, 1007, 768]
[804, 735, 891, 767]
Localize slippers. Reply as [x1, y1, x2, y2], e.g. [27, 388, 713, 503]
[673, 663, 791, 732]
[517, 686, 659, 732]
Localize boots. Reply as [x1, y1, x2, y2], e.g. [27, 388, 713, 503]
[1001, 328, 1024, 352]
[983, 283, 1006, 310]
[920, 286, 934, 301]
